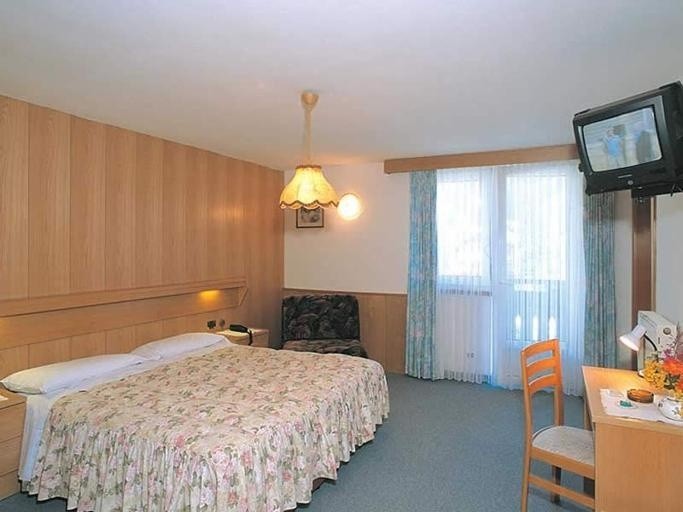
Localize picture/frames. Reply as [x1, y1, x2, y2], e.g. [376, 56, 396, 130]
[296, 207, 324, 229]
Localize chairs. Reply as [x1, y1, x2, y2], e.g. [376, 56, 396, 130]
[519, 336, 594, 512]
[280, 292, 363, 358]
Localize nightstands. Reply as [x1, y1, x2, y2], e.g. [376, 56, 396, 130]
[216, 327, 270, 348]
[0, 386, 26, 500]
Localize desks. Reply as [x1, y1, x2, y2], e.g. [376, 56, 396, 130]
[581, 363, 682, 512]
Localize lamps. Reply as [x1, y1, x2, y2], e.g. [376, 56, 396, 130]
[278, 91, 340, 212]
[619, 325, 658, 378]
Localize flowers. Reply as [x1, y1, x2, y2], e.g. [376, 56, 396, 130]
[644, 323, 683, 400]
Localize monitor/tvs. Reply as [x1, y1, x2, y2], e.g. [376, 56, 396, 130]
[572, 79, 683, 199]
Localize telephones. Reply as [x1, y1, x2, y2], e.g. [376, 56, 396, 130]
[230, 324, 248, 332]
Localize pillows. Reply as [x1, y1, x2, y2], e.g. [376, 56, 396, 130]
[0, 332, 224, 395]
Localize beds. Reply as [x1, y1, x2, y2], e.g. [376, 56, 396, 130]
[14, 343, 389, 512]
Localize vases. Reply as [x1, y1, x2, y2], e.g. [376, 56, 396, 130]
[656, 396, 683, 421]
